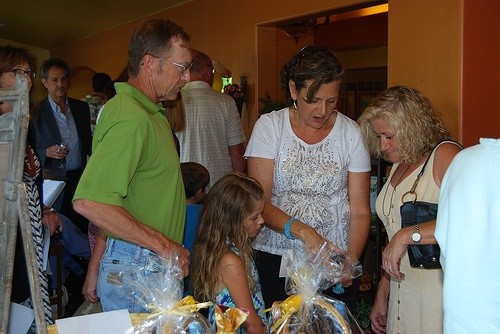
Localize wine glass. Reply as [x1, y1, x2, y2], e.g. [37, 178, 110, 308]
[55, 142, 70, 169]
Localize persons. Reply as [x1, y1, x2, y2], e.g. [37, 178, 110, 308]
[435, 138, 500, 334]
[72, 22, 265, 334]
[27, 59, 93, 239]
[359, 86, 465, 334]
[244, 46, 372, 321]
[0, 45, 63, 307]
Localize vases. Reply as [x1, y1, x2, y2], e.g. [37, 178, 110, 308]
[235, 100, 243, 118]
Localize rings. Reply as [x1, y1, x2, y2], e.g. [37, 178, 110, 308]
[387, 266, 392, 271]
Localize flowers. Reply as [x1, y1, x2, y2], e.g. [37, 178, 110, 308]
[223, 77, 249, 103]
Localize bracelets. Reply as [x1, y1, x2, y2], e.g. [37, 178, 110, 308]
[283, 216, 300, 239]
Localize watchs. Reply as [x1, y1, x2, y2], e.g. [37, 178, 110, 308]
[412, 223, 422, 245]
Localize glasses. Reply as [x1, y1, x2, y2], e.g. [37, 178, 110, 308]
[11, 67, 36, 78]
[148, 51, 194, 72]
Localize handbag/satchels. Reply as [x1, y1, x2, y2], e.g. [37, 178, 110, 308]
[399, 141, 465, 270]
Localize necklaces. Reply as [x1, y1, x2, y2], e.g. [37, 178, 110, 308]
[383, 156, 417, 216]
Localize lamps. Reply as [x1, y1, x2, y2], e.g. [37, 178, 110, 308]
[220, 75, 233, 94]
